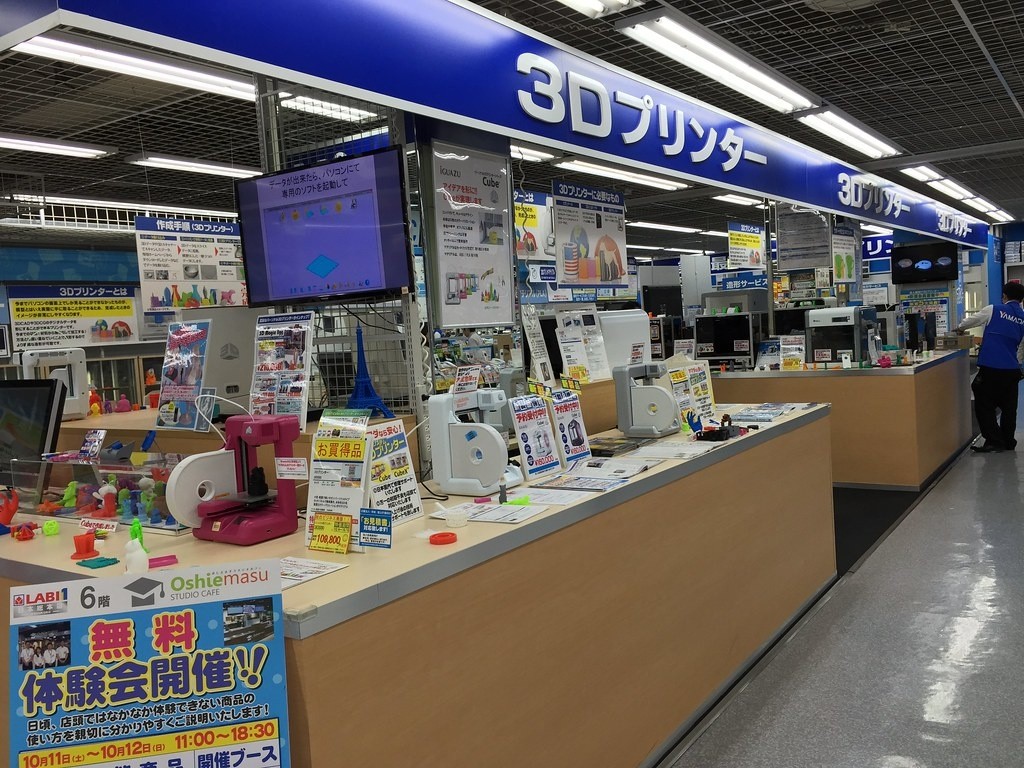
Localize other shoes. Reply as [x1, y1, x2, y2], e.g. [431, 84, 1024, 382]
[970, 440, 1002, 452]
[1003, 439, 1018, 451]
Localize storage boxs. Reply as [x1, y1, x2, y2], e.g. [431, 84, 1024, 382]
[934, 335, 976, 350]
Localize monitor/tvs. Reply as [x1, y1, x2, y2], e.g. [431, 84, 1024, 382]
[519, 313, 564, 384]
[233, 143, 417, 310]
[0, 377, 68, 499]
[890, 241, 958, 284]
[902, 310, 936, 354]
[693, 312, 752, 359]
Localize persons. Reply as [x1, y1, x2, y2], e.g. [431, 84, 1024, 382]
[951, 282, 1024, 453]
[460, 328, 487, 363]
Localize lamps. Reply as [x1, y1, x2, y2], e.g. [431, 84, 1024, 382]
[537, 0, 1013, 221]
